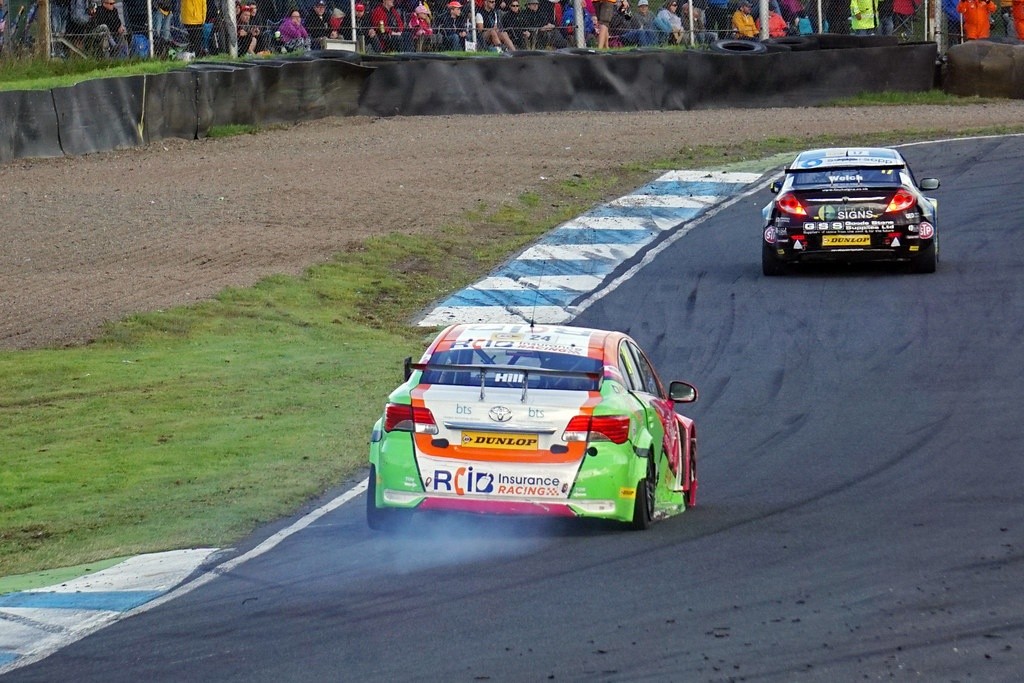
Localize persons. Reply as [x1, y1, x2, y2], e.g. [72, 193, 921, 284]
[0, 0, 1024, 56]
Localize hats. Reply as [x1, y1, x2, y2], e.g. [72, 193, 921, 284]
[240, 5, 253, 12]
[415, 6, 428, 14]
[739, 1, 753, 7]
[448, 1, 463, 9]
[637, 0, 648, 7]
[314, 0, 327, 7]
[527, 0, 539, 3]
[356, 4, 364, 11]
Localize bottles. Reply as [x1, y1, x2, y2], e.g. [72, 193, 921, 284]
[379, 20, 386, 34]
[275, 28, 282, 43]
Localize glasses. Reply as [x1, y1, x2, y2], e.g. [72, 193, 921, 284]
[672, 4, 677, 7]
[105, 2, 115, 5]
[488, 1, 494, 3]
[512, 5, 519, 7]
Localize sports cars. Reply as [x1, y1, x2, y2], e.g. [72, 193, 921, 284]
[761, 145, 940, 274]
[365, 323, 700, 530]
